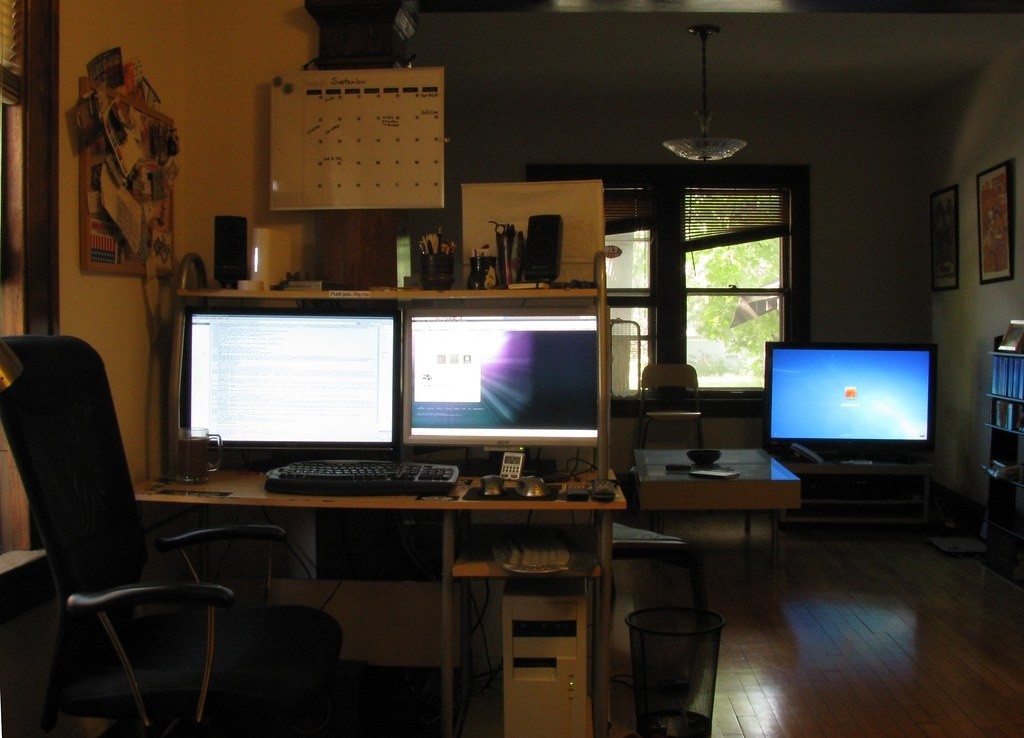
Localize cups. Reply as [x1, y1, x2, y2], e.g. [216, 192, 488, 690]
[422, 252, 454, 291]
[174, 428, 223, 483]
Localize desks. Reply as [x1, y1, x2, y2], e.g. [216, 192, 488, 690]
[631, 448, 801, 534]
[134, 469, 628, 738]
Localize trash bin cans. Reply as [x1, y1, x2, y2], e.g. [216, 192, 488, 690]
[625, 604, 724, 738]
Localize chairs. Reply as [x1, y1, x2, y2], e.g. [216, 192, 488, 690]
[637, 363, 704, 449]
[0, 335, 342, 738]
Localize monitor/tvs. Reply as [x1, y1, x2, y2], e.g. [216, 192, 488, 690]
[765, 342, 939, 462]
[181, 306, 400, 473]
[400, 309, 599, 484]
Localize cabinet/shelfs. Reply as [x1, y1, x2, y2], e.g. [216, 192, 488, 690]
[984, 334, 1024, 588]
[779, 451, 935, 536]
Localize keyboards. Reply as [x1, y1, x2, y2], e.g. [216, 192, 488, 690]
[266, 460, 460, 496]
[492, 536, 577, 573]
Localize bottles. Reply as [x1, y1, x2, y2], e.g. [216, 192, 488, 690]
[467, 256, 499, 289]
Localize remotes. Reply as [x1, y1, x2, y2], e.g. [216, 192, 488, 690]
[566, 483, 589, 502]
[590, 479, 616, 501]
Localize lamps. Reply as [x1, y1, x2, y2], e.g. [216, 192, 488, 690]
[662, 24, 749, 166]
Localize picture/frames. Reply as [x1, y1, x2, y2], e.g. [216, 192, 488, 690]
[998, 319, 1024, 353]
[976, 160, 1014, 286]
[929, 183, 959, 292]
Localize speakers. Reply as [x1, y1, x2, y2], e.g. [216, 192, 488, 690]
[214, 216, 252, 290]
[525, 215, 565, 285]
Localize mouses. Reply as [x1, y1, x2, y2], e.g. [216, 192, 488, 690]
[516, 475, 550, 496]
[479, 475, 507, 496]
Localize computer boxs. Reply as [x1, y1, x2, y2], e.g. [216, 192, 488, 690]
[501, 577, 591, 738]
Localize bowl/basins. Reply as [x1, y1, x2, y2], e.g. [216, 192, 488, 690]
[687, 450, 721, 465]
